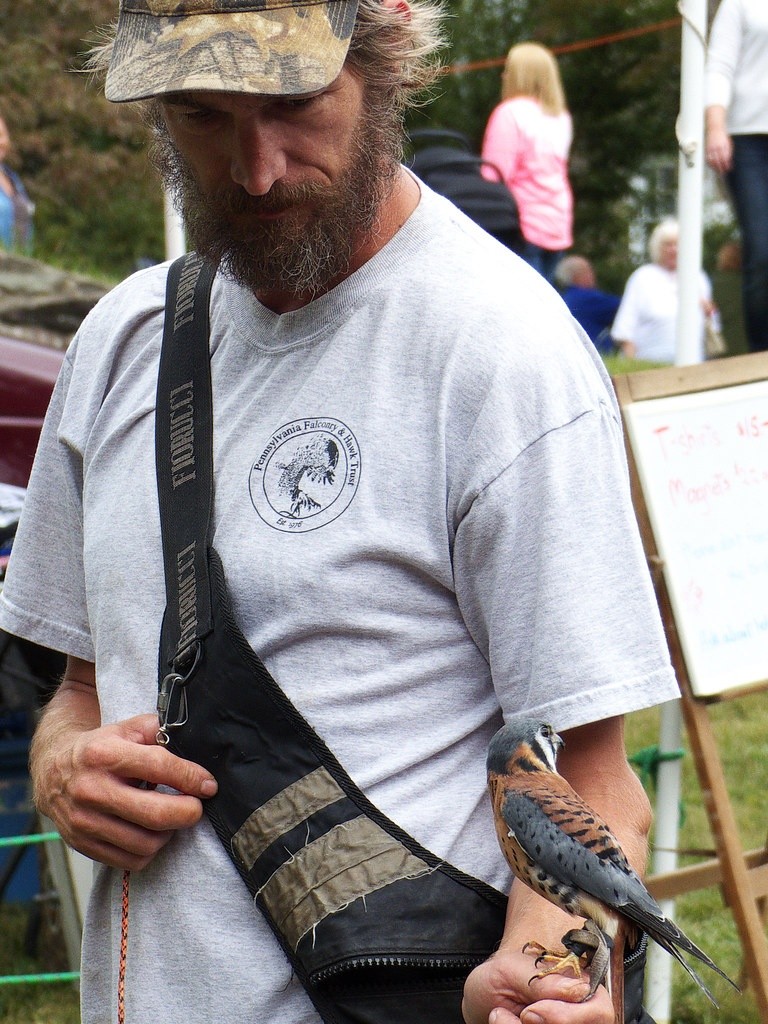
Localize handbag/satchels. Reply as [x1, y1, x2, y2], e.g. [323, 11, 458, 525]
[157, 546, 650, 1024]
[703, 327, 726, 357]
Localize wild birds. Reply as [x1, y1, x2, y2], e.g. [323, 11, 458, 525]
[486, 717, 743, 1011]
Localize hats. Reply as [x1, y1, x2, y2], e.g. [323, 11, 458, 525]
[104, 0, 363, 103]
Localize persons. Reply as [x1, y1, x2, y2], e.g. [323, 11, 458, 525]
[1, 118, 37, 255]
[2, 0, 681, 1024]
[484, 40, 733, 366]
[702, 1, 768, 351]
